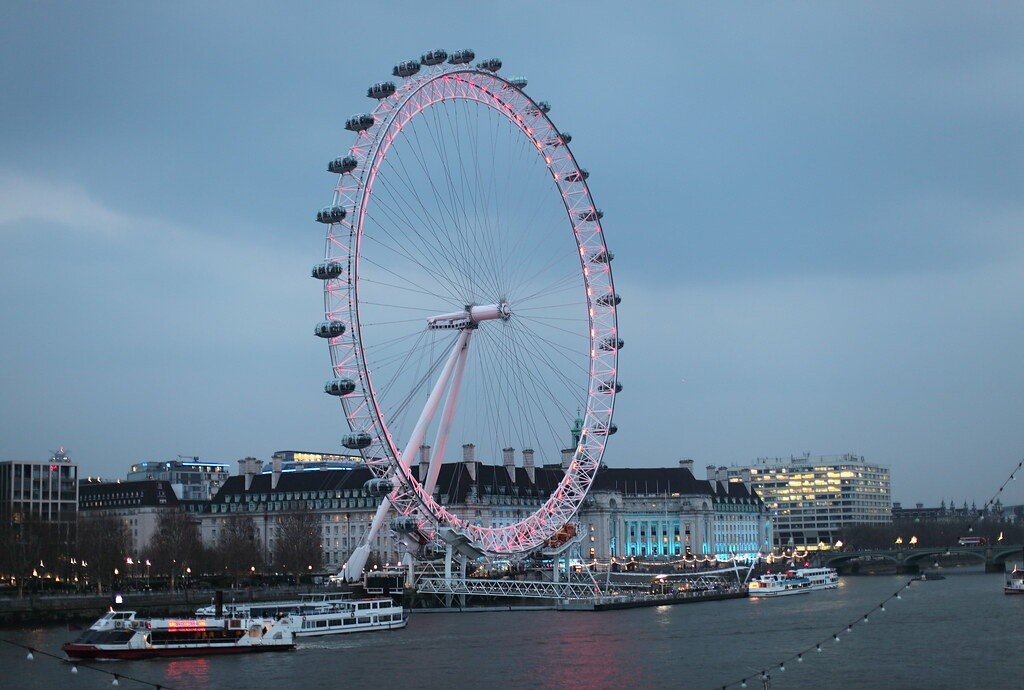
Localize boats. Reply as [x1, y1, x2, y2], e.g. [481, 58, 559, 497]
[749, 574, 812, 597]
[789, 563, 839, 590]
[63, 611, 296, 659]
[1004, 564, 1024, 594]
[195, 602, 300, 615]
[239, 594, 412, 636]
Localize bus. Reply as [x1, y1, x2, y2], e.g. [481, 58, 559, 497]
[958, 537, 986, 546]
